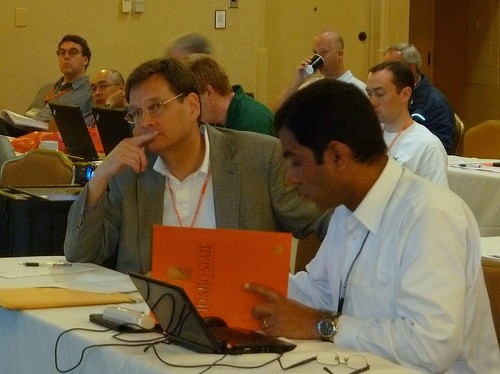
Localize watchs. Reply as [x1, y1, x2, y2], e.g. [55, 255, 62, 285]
[317, 311, 338, 342]
[104, 103, 115, 109]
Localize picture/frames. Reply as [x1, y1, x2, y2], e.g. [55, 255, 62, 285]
[215, 10, 226, 29]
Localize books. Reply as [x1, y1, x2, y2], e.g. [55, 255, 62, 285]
[150, 224, 299, 332]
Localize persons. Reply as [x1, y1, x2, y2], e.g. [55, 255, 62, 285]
[64, 60, 336, 277]
[271, 29, 369, 112]
[86, 69, 129, 129]
[244, 78, 500, 374]
[180, 53, 279, 139]
[0, 34, 92, 170]
[384, 41, 457, 154]
[366, 61, 449, 190]
[162, 31, 209, 64]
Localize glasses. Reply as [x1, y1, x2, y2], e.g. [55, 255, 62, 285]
[56, 48, 83, 57]
[124, 92, 184, 126]
[91, 84, 121, 92]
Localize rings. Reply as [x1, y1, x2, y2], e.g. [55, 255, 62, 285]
[264, 318, 268, 328]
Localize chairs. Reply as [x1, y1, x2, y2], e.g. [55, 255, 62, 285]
[453, 113, 465, 155]
[0, 149, 75, 187]
[460, 120, 500, 159]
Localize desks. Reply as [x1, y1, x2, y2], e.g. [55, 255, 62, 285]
[446, 155, 500, 238]
[0, 256, 420, 374]
[0, 185, 87, 258]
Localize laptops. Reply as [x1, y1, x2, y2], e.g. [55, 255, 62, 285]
[91, 106, 135, 157]
[48, 102, 99, 161]
[127, 271, 297, 354]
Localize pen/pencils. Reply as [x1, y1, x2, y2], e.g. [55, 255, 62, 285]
[459, 164, 482, 168]
[19, 262, 72, 267]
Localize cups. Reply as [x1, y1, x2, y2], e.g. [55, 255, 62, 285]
[305, 54, 326, 75]
